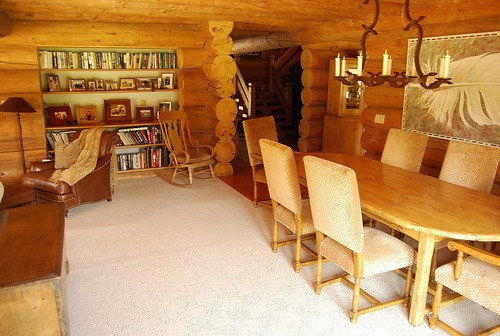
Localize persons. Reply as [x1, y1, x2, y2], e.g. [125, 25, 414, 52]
[98, 81, 102, 88]
[87, 111, 91, 120]
[50, 77, 58, 89]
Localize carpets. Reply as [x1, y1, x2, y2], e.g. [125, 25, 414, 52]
[60, 169, 500, 336]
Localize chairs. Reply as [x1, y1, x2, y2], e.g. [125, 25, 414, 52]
[158, 111, 216, 188]
[257, 137, 330, 272]
[430, 140, 500, 285]
[427, 239, 500, 336]
[302, 154, 418, 324]
[367, 127, 430, 238]
[240, 115, 279, 209]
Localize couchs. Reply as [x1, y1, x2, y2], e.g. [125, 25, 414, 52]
[0, 181, 66, 290]
[20, 127, 119, 218]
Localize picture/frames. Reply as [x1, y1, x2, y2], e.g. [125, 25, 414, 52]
[46, 105, 75, 126]
[46, 74, 62, 92]
[136, 107, 154, 122]
[96, 79, 105, 91]
[68, 79, 86, 92]
[120, 78, 135, 90]
[104, 99, 132, 123]
[136, 77, 153, 91]
[105, 82, 119, 90]
[75, 104, 99, 125]
[157, 102, 172, 120]
[87, 80, 97, 91]
[161, 73, 174, 89]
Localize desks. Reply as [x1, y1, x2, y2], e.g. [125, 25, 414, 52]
[291, 150, 500, 331]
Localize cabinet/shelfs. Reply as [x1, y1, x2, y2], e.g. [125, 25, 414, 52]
[322, 57, 366, 158]
[37, 46, 184, 174]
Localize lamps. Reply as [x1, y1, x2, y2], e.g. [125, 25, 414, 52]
[332, 0, 454, 90]
[0, 96, 38, 174]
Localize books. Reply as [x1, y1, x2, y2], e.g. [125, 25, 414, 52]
[38, 50, 176, 69]
[45, 131, 77, 149]
[114, 127, 186, 171]
